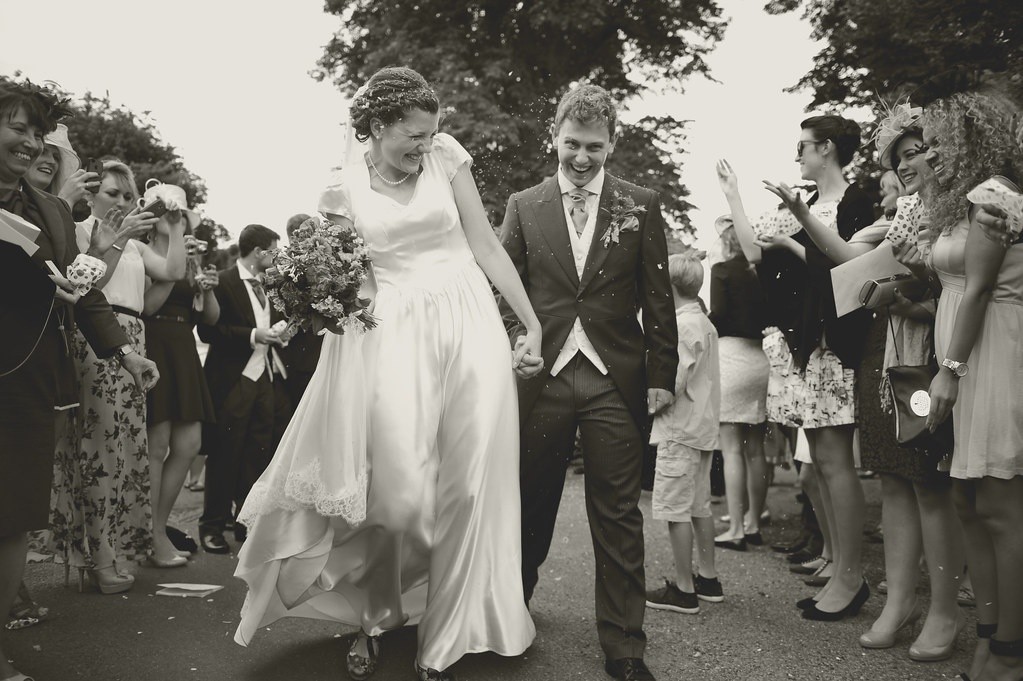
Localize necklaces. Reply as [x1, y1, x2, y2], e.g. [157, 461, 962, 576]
[367, 147, 415, 185]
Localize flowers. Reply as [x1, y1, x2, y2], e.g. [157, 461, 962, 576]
[599, 194, 647, 250]
[265, 217, 380, 338]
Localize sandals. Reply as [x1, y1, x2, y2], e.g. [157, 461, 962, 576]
[957, 570, 976, 604]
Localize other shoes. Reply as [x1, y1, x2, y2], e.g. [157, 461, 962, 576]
[413, 658, 456, 681]
[185, 481, 204, 491]
[11, 581, 48, 622]
[346, 626, 380, 678]
[772, 527, 836, 585]
[714, 528, 761, 551]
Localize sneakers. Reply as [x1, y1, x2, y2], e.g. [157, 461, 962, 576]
[645, 571, 724, 615]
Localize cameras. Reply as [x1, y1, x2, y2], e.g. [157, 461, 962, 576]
[187, 239, 208, 255]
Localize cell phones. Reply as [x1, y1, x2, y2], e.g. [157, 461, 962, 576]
[136, 198, 168, 218]
[84, 157, 103, 193]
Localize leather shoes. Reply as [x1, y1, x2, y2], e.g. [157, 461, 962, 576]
[200, 532, 229, 553]
[605, 658, 656, 681]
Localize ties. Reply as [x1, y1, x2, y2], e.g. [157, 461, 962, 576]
[252, 279, 267, 310]
[567, 188, 589, 238]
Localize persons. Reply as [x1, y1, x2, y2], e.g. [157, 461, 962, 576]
[0, 74, 360, 681]
[236, 65, 545, 681]
[494, 85, 680, 681]
[645, 254, 725, 615]
[709, 90, 1023, 681]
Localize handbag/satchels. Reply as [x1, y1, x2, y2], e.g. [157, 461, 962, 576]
[885, 361, 954, 444]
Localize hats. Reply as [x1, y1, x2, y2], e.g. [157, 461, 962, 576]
[714, 212, 761, 236]
[43, 124, 82, 191]
[139, 183, 201, 230]
[908, 66, 969, 105]
[878, 106, 927, 170]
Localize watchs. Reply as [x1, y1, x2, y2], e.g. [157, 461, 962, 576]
[116, 345, 135, 356]
[941, 358, 969, 379]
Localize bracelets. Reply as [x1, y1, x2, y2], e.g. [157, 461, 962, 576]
[110, 244, 124, 254]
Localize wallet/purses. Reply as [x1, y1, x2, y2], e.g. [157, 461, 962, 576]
[859, 274, 922, 309]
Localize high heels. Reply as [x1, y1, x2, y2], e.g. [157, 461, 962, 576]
[145, 542, 192, 567]
[61, 545, 135, 594]
[797, 576, 870, 622]
[860, 595, 969, 660]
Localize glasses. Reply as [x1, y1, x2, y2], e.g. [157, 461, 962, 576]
[796, 136, 829, 156]
